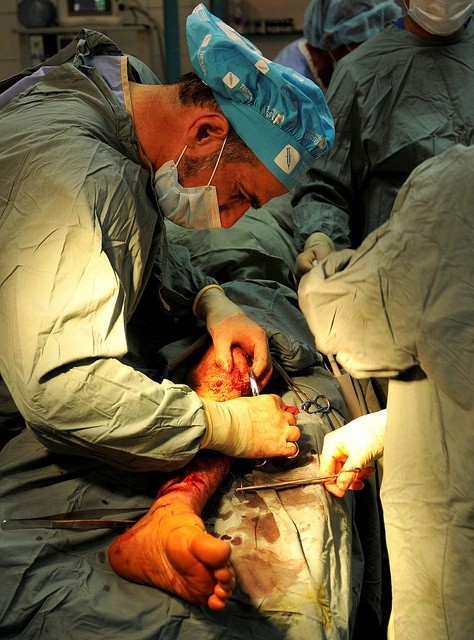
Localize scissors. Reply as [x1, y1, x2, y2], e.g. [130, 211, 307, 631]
[270, 356, 332, 412]
[249, 372, 299, 467]
[237, 468, 362, 492]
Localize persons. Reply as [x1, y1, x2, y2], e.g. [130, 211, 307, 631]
[294, 1, 473, 279]
[0, 0, 335, 462]
[298, 146, 474, 640]
[107, 347, 252, 612]
[270, 2, 401, 98]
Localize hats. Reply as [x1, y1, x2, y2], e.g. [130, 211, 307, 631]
[186, 2, 335, 193]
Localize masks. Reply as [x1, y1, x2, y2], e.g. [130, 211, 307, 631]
[153, 134, 222, 231]
[407, 0, 473, 36]
